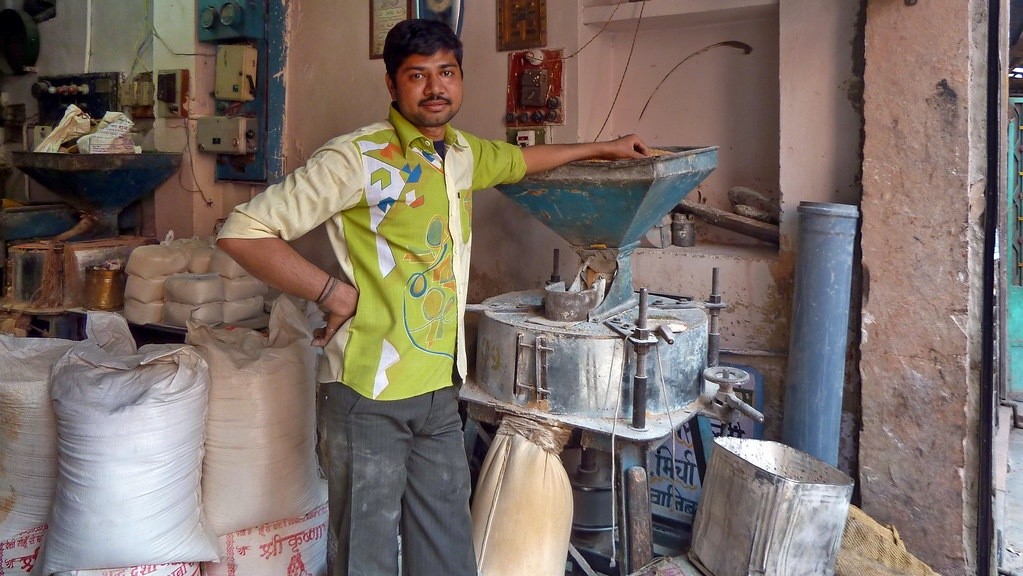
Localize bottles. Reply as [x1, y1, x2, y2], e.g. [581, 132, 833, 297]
[672, 213, 696, 246]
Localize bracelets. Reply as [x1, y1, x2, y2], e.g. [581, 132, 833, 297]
[315, 274, 338, 305]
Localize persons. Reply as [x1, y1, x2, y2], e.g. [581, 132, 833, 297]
[215, 19, 649, 576]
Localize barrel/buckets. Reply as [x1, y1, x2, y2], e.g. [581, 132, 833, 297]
[687, 435, 856, 576]
[84, 265, 127, 311]
